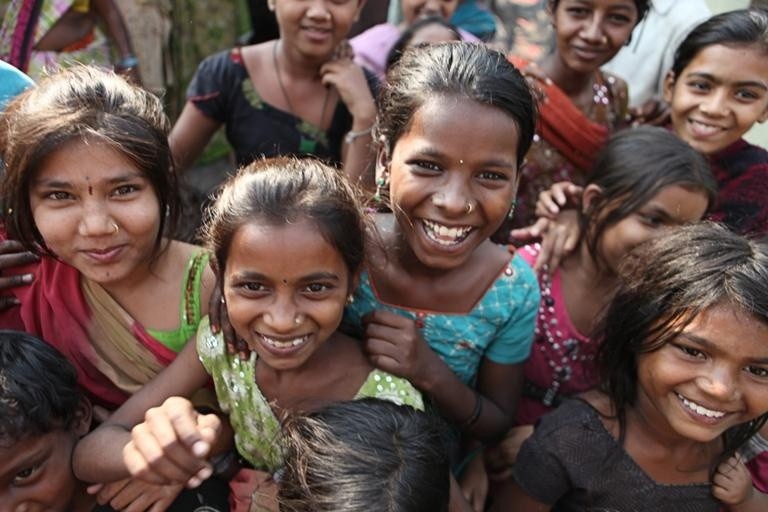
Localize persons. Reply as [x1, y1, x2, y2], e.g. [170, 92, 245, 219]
[0, 0, 768, 512]
